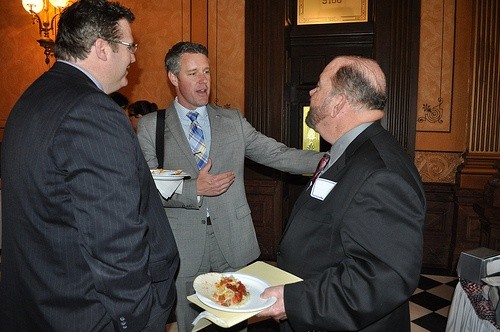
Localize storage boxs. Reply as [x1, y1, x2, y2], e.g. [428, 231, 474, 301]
[456, 247, 500, 285]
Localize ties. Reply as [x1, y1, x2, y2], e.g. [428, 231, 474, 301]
[186, 110, 210, 218]
[305, 154, 330, 191]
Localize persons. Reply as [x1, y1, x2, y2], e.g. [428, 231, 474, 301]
[0, 2, 180, 332]
[137, 42, 324, 332]
[110, 92, 158, 125]
[256, 56, 426, 332]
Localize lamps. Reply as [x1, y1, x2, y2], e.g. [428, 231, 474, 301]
[21, 0, 71, 65]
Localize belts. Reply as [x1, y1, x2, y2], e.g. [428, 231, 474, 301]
[207, 217, 212, 225]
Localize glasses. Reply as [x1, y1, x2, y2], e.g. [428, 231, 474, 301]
[129, 112, 137, 117]
[99, 35, 137, 53]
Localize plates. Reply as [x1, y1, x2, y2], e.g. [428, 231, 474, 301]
[196, 272, 278, 314]
[150, 169, 190, 180]
